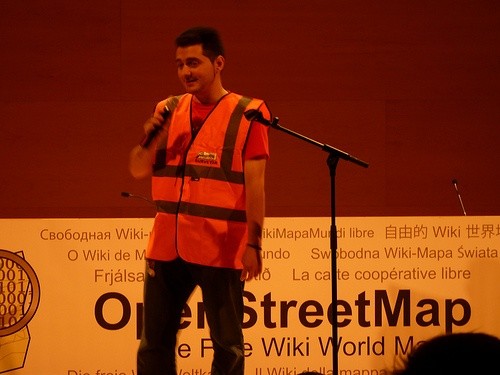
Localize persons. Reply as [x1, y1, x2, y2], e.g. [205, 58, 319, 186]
[388, 332, 500, 375]
[129, 26, 270, 375]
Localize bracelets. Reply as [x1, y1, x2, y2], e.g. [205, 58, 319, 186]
[246, 243, 262, 251]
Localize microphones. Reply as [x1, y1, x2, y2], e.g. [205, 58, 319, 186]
[452, 178, 466, 216]
[122, 192, 151, 205]
[139, 95, 178, 147]
